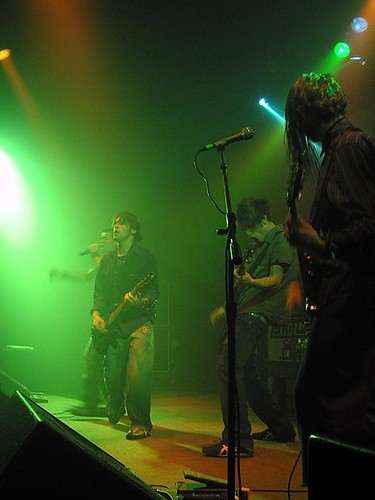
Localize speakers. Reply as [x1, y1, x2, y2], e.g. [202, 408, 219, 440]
[0, 370, 31, 401]
[0, 389, 165, 500]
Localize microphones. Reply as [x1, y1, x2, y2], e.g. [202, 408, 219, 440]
[199, 127, 255, 152]
[79, 243, 104, 256]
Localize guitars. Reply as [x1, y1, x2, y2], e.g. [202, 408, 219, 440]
[285, 180, 321, 323]
[219, 237, 260, 345]
[91, 271, 157, 354]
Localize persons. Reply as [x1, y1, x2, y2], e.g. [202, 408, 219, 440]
[281, 70, 375, 500]
[50, 227, 126, 418]
[202, 197, 299, 457]
[90, 211, 161, 440]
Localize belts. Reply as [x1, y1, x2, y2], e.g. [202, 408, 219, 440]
[248, 312, 269, 329]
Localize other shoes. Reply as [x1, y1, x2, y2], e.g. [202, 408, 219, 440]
[126, 425, 150, 440]
[69, 405, 98, 415]
[109, 406, 126, 425]
[100, 405, 126, 416]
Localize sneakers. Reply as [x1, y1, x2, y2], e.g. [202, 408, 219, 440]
[251, 428, 296, 442]
[201, 441, 254, 457]
